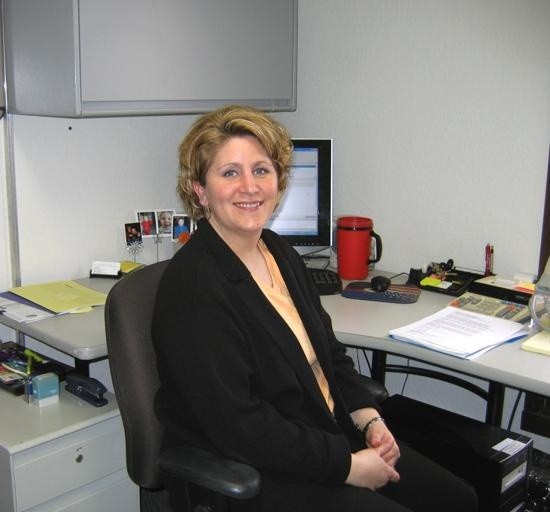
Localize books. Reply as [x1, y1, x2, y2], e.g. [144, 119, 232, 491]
[388, 305, 530, 363]
[8, 279, 109, 316]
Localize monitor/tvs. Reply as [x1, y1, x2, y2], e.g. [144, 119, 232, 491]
[264, 137, 333, 264]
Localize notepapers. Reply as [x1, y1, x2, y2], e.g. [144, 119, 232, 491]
[520, 330, 550, 356]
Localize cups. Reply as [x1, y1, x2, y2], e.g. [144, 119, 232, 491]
[336, 215, 382, 280]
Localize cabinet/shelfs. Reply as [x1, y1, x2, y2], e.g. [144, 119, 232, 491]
[3, 1, 298, 118]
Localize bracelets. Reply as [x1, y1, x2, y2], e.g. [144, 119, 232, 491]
[362, 416, 385, 434]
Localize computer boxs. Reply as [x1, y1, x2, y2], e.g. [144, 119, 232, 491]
[380, 392, 534, 512]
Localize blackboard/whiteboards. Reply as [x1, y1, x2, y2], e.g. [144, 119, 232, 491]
[72, 0, 299, 117]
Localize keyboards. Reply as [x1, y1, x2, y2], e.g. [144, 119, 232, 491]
[307, 268, 343, 295]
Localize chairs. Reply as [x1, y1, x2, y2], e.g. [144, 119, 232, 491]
[104, 258, 263, 511]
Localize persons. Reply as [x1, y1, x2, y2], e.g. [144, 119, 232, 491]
[141, 215, 154, 235]
[127, 226, 132, 241]
[149, 101, 485, 512]
[174, 218, 188, 238]
[158, 211, 172, 234]
[131, 227, 141, 242]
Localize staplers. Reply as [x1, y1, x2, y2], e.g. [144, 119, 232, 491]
[64, 373, 108, 407]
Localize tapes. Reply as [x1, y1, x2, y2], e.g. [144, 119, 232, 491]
[422, 262, 434, 274]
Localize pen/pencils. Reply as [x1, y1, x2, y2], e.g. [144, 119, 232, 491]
[486, 242, 494, 275]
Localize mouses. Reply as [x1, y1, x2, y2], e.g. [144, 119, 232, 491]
[371, 276, 391, 292]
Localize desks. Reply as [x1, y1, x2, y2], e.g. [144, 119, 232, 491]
[1, 262, 550, 512]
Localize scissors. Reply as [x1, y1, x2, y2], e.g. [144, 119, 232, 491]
[439, 259, 454, 272]
